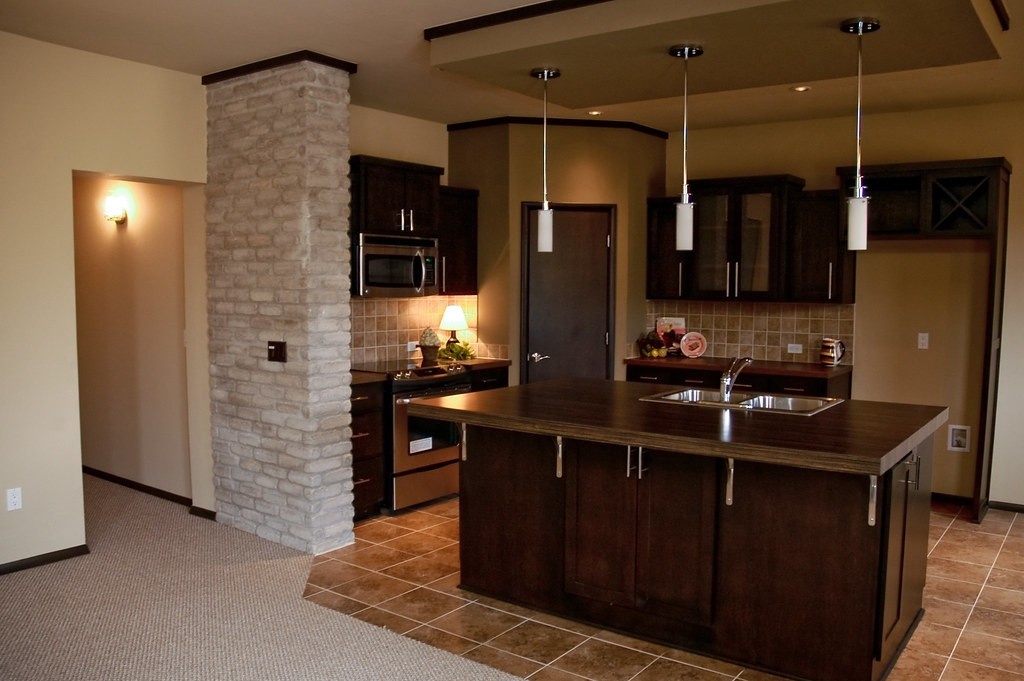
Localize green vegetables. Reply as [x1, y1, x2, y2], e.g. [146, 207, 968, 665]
[438, 342, 476, 360]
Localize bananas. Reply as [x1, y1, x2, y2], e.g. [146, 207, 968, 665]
[642, 344, 652, 358]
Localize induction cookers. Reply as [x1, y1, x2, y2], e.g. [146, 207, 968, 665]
[350, 358, 467, 393]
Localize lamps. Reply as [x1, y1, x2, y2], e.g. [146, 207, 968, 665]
[669, 44, 702, 251]
[841, 17, 883, 252]
[529, 67, 562, 253]
[440, 305, 467, 346]
[104, 196, 127, 225]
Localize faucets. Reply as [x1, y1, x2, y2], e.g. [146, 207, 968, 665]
[719, 357, 754, 403]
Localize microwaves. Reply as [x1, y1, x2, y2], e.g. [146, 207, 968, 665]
[351, 232, 439, 298]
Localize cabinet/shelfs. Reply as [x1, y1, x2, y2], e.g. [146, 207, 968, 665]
[471, 367, 508, 390]
[351, 371, 392, 521]
[561, 438, 714, 657]
[683, 177, 786, 304]
[441, 187, 479, 295]
[647, 197, 684, 298]
[356, 155, 445, 238]
[625, 365, 852, 399]
[875, 434, 934, 664]
[836, 156, 1013, 242]
[788, 189, 843, 304]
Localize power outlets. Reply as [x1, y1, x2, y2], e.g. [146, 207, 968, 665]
[918, 332, 929, 349]
[787, 344, 802, 354]
[408, 341, 419, 351]
[8, 488, 22, 511]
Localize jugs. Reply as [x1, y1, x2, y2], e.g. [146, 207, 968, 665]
[820, 338, 846, 367]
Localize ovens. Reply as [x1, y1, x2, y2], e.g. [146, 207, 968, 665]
[382, 384, 472, 512]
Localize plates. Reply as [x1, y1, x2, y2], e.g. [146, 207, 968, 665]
[680, 331, 708, 359]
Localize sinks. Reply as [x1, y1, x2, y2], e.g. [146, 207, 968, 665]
[638, 386, 757, 409]
[732, 392, 845, 415]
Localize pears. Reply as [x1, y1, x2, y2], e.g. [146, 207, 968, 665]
[651, 347, 667, 357]
